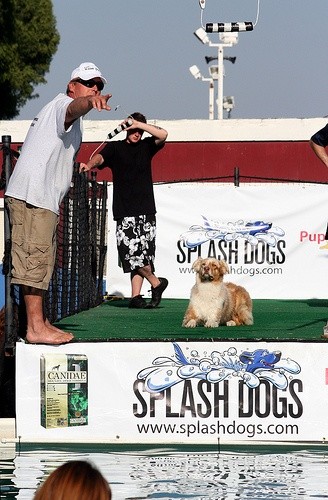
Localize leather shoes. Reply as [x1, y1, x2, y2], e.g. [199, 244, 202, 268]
[146, 277, 169, 310]
[128, 294, 148, 308]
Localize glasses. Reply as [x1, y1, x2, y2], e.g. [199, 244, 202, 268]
[71, 79, 104, 91]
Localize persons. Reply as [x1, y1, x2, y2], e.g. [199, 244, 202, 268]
[310, 124, 328, 338]
[5, 62, 112, 345]
[33, 461, 111, 500]
[79, 112, 168, 309]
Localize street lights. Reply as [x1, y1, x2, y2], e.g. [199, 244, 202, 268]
[192, 26, 239, 119]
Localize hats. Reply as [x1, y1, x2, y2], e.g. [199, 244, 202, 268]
[69, 62, 108, 84]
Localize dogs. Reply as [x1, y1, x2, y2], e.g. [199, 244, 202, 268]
[181, 256, 254, 329]
